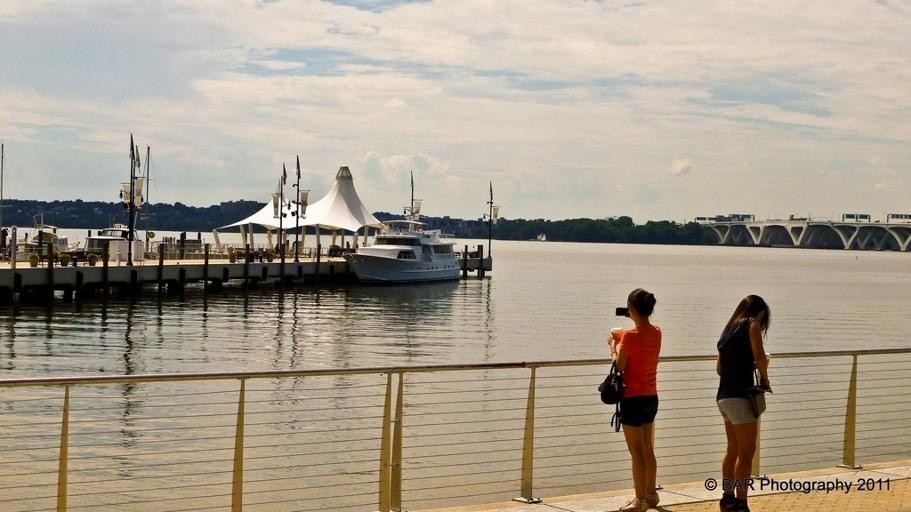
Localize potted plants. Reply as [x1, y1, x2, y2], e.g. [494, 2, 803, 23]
[266, 253, 275, 262]
[58, 254, 71, 267]
[229, 253, 237, 263]
[27, 253, 40, 268]
[86, 254, 99, 266]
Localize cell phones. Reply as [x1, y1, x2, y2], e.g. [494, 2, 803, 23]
[616, 308, 628, 315]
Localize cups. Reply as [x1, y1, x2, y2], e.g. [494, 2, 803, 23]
[611, 328, 625, 345]
[765, 353, 771, 370]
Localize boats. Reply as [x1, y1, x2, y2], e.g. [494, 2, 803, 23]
[354, 219, 462, 284]
[72, 223, 146, 261]
[12, 224, 70, 262]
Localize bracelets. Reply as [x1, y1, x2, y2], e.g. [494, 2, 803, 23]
[611, 352, 616, 357]
[761, 379, 768, 382]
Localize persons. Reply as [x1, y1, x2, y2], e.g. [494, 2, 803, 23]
[598, 288, 662, 512]
[715, 295, 773, 512]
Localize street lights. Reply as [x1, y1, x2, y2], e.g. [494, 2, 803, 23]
[118, 190, 135, 266]
[278, 199, 292, 264]
[290, 181, 302, 262]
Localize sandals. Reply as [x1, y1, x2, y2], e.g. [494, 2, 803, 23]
[617, 492, 659, 512]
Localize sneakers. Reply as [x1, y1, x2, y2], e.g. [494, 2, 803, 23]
[719, 493, 750, 512]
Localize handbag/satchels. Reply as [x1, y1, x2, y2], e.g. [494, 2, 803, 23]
[597, 359, 623, 405]
[749, 383, 766, 419]
